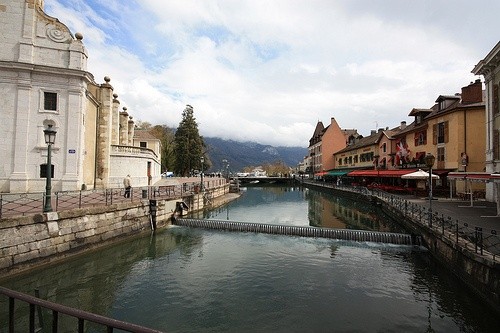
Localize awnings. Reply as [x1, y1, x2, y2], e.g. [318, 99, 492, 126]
[379, 168, 416, 177]
[323, 170, 352, 176]
[347, 169, 378, 177]
[489, 174, 500, 217]
[448, 170, 491, 207]
[302, 170, 329, 176]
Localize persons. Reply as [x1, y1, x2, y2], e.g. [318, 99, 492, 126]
[149, 175, 152, 185]
[123, 174, 132, 197]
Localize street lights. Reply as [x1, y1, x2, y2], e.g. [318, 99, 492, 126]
[376, 166, 380, 196]
[200, 156, 206, 190]
[424, 152, 436, 226]
[43, 117, 57, 214]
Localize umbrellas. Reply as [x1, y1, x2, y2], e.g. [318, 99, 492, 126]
[401, 169, 439, 181]
